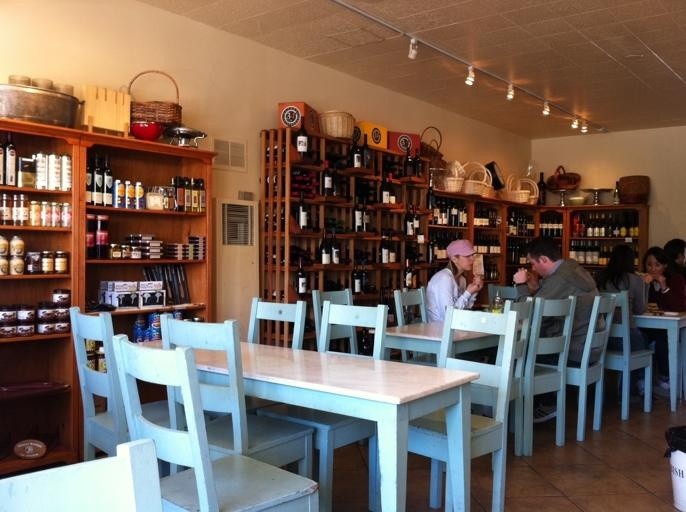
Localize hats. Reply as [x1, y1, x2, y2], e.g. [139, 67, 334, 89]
[446, 238, 477, 258]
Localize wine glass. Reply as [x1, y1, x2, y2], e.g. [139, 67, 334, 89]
[581, 187, 614, 205]
[552, 189, 576, 207]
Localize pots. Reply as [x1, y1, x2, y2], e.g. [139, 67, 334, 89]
[0, 83, 87, 129]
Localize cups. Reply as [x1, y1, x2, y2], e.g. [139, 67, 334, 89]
[7, 75, 73, 96]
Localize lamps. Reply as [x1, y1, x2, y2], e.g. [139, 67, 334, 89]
[402, 32, 592, 135]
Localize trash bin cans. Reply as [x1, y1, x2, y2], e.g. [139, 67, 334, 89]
[664, 425, 686, 512]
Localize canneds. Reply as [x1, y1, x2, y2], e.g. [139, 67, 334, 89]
[108, 244, 142, 260]
[1, 194, 71, 227]
[0, 235, 68, 275]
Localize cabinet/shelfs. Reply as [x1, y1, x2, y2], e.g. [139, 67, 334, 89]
[0, 114, 221, 480]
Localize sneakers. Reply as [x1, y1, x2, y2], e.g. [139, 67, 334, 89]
[658, 375, 670, 391]
[533, 403, 557, 424]
[617, 396, 642, 408]
[636, 378, 645, 396]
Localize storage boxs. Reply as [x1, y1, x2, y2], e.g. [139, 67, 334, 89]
[352, 118, 389, 150]
[277, 100, 321, 134]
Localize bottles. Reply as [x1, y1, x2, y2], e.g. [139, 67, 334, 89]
[614, 189, 620, 204]
[506, 208, 563, 288]
[114, 178, 146, 210]
[568, 209, 639, 279]
[0, 130, 18, 187]
[170, 175, 206, 214]
[86, 146, 114, 207]
[492, 288, 502, 314]
[24, 249, 68, 274]
[31, 150, 72, 191]
[426, 174, 503, 288]
[535, 171, 547, 206]
[0, 193, 72, 228]
[0, 234, 25, 276]
[260, 114, 423, 356]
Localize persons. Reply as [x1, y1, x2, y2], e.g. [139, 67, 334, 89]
[425, 239, 483, 323]
[664, 240, 685, 268]
[596, 245, 651, 395]
[644, 246, 686, 391]
[513, 237, 608, 423]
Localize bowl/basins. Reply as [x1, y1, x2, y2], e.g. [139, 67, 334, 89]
[569, 196, 587, 205]
[131, 120, 166, 141]
